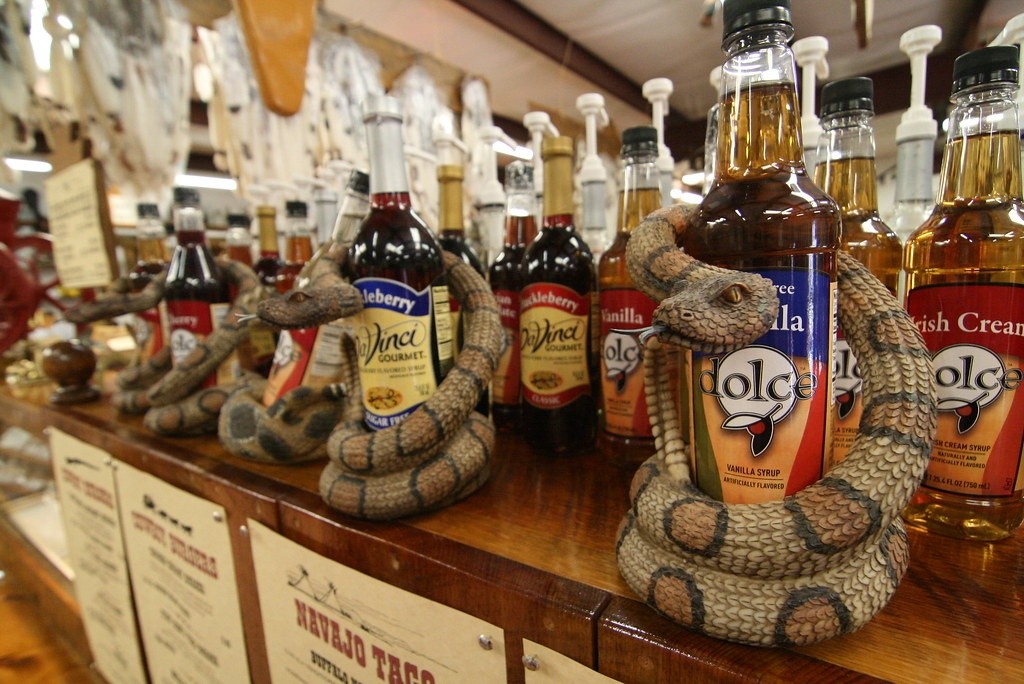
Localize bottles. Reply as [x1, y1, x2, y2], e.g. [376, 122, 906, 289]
[126, 0, 1024, 542]
